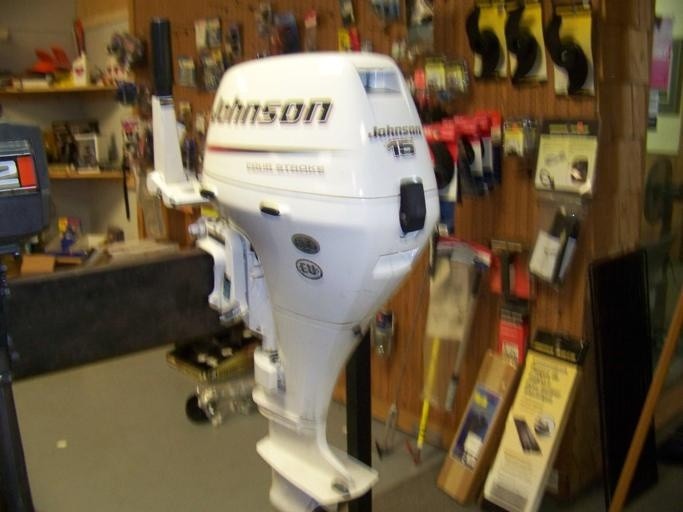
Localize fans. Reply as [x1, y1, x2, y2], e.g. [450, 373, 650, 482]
[642, 158, 675, 352]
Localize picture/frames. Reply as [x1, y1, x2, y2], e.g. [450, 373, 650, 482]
[658, 40, 683, 112]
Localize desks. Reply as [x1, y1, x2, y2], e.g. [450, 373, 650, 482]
[0, 235, 213, 381]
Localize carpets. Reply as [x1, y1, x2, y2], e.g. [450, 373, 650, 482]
[11, 341, 450, 512]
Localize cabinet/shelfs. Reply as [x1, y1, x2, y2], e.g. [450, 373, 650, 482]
[0, 0, 146, 240]
